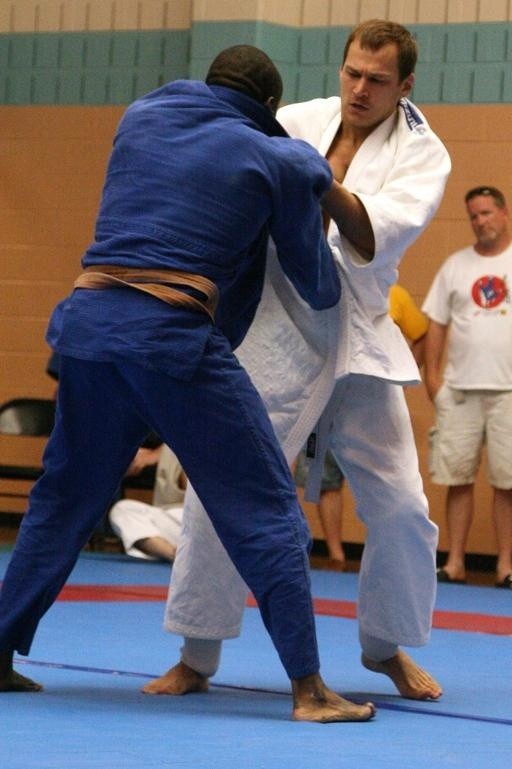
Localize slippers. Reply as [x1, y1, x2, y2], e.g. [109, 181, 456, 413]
[495, 574, 511, 588]
[435, 567, 467, 583]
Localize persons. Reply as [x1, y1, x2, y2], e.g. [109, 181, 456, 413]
[421, 184, 512, 586]
[76, 433, 165, 551]
[142, 19, 445, 701]
[309, 280, 429, 562]
[107, 444, 187, 561]
[2, 46, 376, 722]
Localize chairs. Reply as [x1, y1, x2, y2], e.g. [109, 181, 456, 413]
[0, 399, 56, 528]
